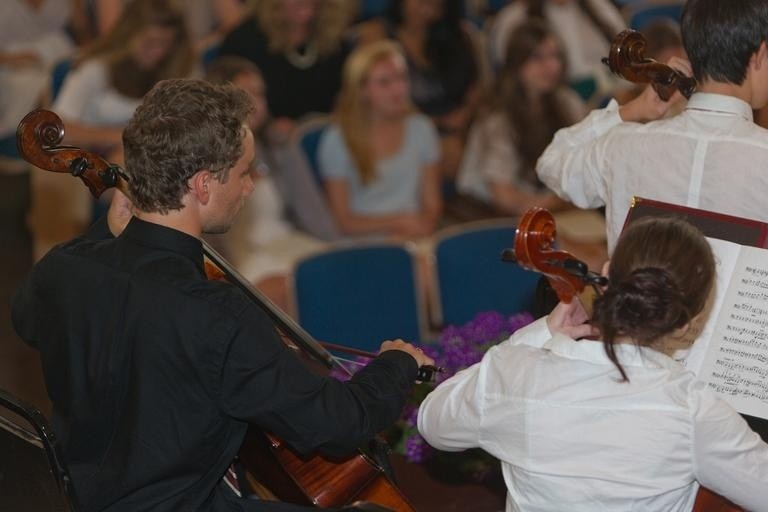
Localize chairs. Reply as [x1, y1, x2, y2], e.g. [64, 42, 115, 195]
[293, 118, 330, 199]
[39, 47, 75, 110]
[295, 238, 424, 362]
[0, 133, 21, 160]
[620, 1, 686, 34]
[2, 391, 82, 511]
[429, 114, 458, 199]
[424, 215, 547, 331]
[193, 34, 220, 68]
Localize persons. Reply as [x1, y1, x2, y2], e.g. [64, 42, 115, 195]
[536, 0, 768, 258]
[416, 217, 768, 511]
[2, 1, 695, 281]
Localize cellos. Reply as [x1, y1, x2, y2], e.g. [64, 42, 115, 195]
[601, 26, 765, 126]
[501, 208, 742, 509]
